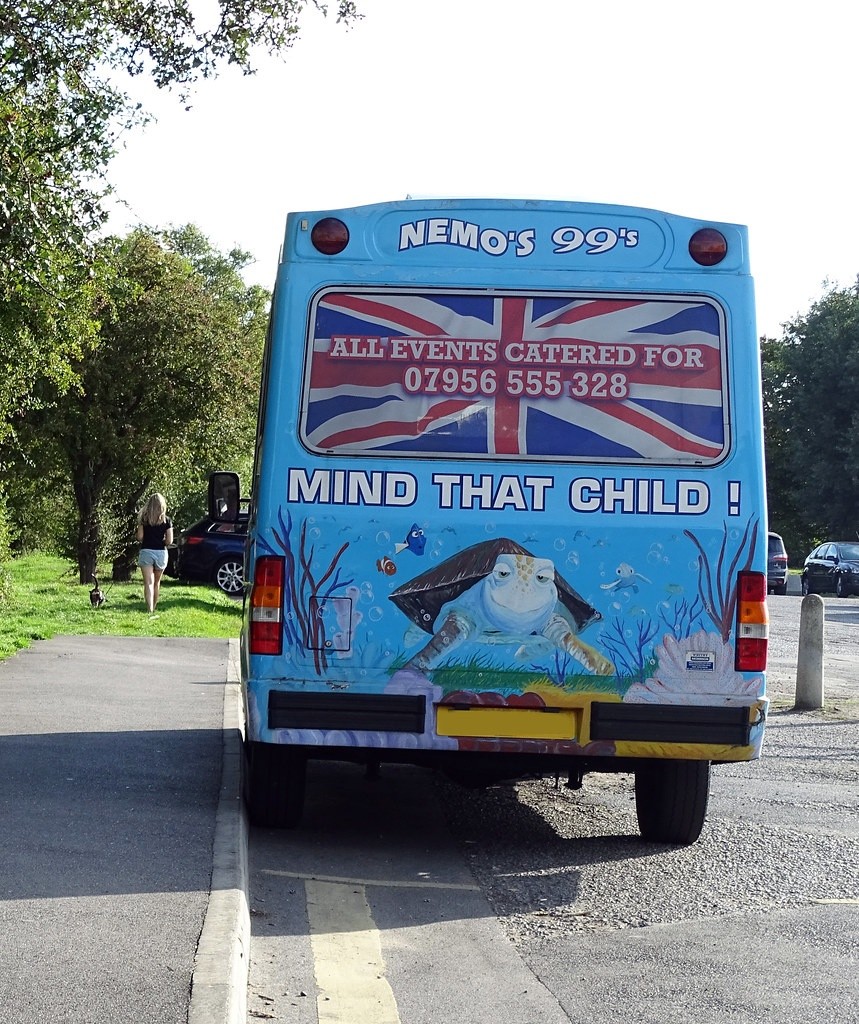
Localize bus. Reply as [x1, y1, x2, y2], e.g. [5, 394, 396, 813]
[207, 195, 770, 844]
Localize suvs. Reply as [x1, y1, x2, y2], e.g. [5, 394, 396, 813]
[176, 470, 251, 599]
[766, 531, 788, 596]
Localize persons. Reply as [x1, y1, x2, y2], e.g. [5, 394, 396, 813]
[136, 493, 173, 614]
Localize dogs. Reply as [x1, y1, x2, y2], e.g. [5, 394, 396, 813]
[89, 574, 105, 608]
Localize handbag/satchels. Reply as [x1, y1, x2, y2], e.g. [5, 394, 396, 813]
[168, 544, 179, 562]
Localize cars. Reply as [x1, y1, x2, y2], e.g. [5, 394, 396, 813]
[801, 541, 859, 599]
[164, 544, 179, 579]
